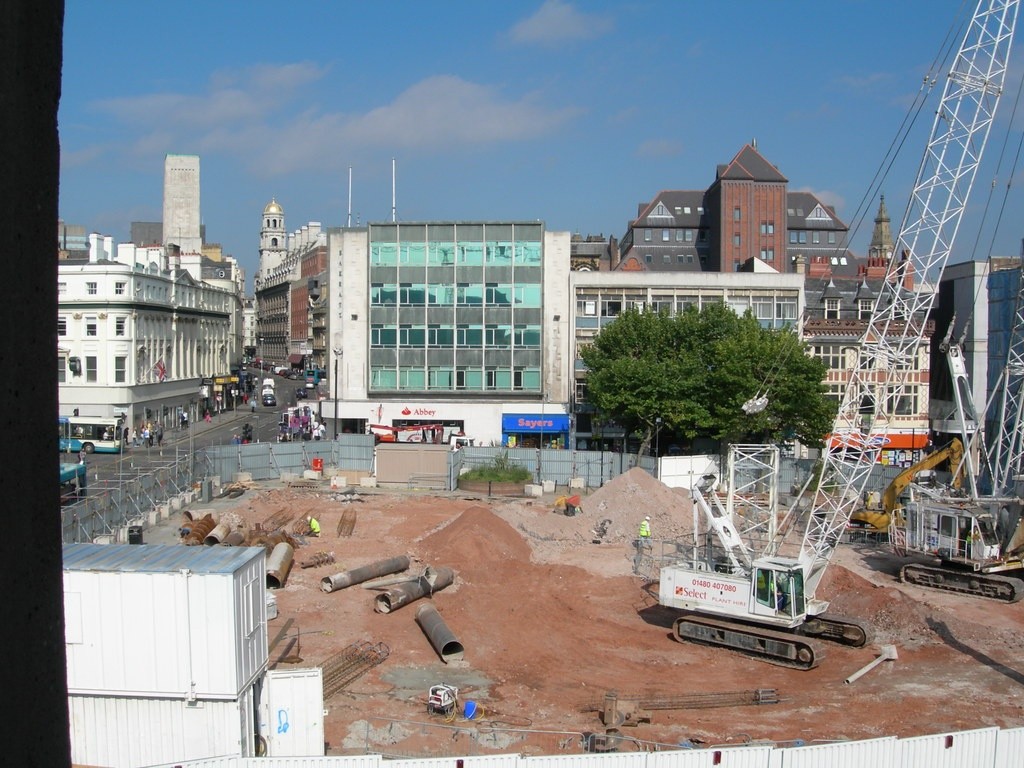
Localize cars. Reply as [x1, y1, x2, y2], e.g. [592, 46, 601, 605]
[248, 360, 303, 380]
[296, 388, 308, 398]
[264, 394, 276, 405]
[305, 381, 315, 389]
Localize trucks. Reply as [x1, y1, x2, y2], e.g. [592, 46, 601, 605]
[370, 423, 474, 451]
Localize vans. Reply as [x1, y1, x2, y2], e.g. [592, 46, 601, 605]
[261, 389, 274, 395]
[263, 378, 275, 388]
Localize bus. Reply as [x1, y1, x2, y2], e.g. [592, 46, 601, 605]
[59, 416, 125, 453]
[303, 369, 326, 384]
[60, 461, 88, 507]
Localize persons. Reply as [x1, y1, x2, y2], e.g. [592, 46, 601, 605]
[102, 429, 113, 440]
[639, 516, 652, 548]
[205, 409, 212, 423]
[179, 410, 189, 430]
[240, 391, 256, 412]
[230, 431, 252, 446]
[279, 412, 326, 442]
[965, 525, 981, 556]
[307, 516, 320, 537]
[59, 424, 83, 438]
[123, 420, 164, 448]
[770, 577, 782, 593]
[79, 447, 86, 464]
[245, 374, 258, 392]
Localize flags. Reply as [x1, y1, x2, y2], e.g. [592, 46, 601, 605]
[152, 358, 167, 384]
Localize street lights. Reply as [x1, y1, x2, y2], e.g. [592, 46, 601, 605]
[253, 415, 260, 443]
[655, 414, 661, 478]
[333, 347, 341, 439]
[255, 319, 264, 378]
[300, 321, 307, 369]
[540, 396, 551, 482]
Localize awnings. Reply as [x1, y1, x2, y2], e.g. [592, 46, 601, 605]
[287, 354, 304, 363]
[823, 433, 928, 450]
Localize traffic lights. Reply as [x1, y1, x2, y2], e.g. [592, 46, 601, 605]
[163, 407, 168, 416]
[294, 410, 299, 418]
[283, 413, 289, 427]
[145, 408, 152, 419]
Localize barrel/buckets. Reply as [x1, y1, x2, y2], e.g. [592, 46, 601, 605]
[464, 701, 475, 719]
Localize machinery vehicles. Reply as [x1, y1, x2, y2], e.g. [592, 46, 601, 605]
[659, 1, 1023, 674]
[890, 244, 1024, 605]
[844, 435, 965, 541]
